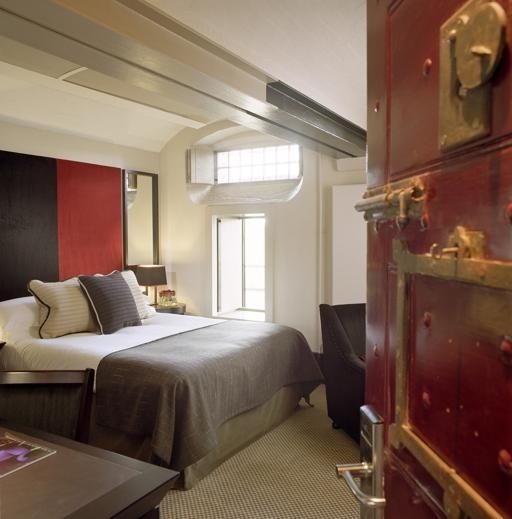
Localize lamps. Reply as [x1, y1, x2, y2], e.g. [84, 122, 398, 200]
[135, 265, 166, 308]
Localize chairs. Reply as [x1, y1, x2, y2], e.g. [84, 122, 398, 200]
[0, 367, 95, 445]
[319, 303, 366, 445]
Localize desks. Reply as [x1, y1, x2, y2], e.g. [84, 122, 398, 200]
[0, 416, 181, 519]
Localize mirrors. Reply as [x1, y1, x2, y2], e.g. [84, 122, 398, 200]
[122, 168, 159, 269]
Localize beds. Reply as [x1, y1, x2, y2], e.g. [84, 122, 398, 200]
[0, 311, 327, 491]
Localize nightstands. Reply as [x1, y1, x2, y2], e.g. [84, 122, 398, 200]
[147, 302, 186, 315]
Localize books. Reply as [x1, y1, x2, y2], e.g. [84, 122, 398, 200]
[0, 435, 57, 480]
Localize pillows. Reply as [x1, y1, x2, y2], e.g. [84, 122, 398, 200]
[0, 296, 43, 343]
[93, 270, 156, 320]
[78, 270, 143, 336]
[26, 276, 98, 339]
